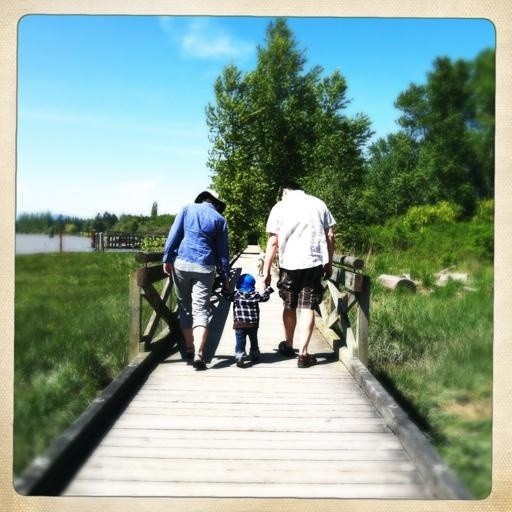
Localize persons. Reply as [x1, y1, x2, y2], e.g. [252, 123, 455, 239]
[262, 181, 337, 368]
[221, 274, 274, 368]
[162, 189, 232, 370]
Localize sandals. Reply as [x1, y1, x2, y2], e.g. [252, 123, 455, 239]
[193, 354, 206, 368]
[186, 347, 194, 358]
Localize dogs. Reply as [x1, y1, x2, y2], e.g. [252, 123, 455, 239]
[256, 253, 266, 276]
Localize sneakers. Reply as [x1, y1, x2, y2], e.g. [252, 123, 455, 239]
[250, 352, 260, 360]
[237, 356, 244, 368]
[298, 354, 316, 368]
[279, 341, 294, 357]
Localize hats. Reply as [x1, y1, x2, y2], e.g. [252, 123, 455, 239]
[237, 274, 255, 293]
[195, 189, 226, 214]
